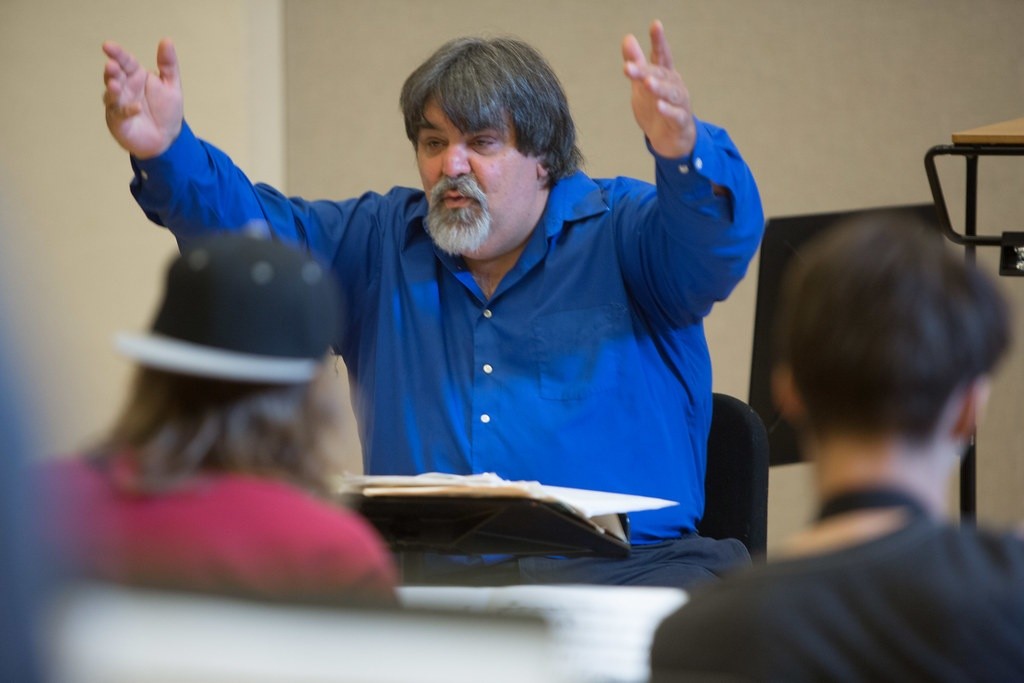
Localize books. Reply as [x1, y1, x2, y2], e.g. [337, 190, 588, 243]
[339, 472, 679, 553]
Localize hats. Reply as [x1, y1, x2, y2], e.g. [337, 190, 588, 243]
[108, 235, 343, 384]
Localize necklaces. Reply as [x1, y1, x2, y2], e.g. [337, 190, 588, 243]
[814, 480, 925, 525]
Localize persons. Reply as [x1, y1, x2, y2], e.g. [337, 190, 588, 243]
[103, 20, 765, 600]
[36, 234, 403, 607]
[650, 220, 1023, 683]
